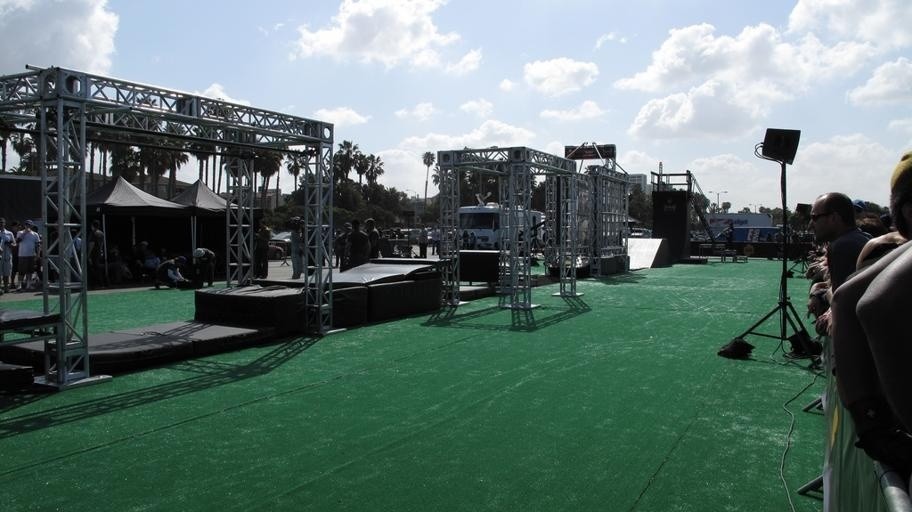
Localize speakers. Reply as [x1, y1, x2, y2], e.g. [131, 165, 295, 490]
[759, 125, 802, 166]
[795, 202, 813, 222]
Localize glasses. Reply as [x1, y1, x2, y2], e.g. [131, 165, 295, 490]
[811, 212, 830, 222]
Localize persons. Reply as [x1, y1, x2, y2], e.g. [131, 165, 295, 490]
[0, 216, 174, 293]
[195, 247, 216, 288]
[468, 232, 476, 249]
[801, 151, 912, 486]
[290, 218, 440, 281]
[154, 256, 189, 290]
[461, 230, 469, 249]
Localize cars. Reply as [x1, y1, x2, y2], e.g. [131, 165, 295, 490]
[269, 232, 293, 256]
[267, 244, 285, 260]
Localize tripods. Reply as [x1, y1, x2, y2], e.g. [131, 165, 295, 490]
[718, 171, 824, 360]
[787, 223, 811, 275]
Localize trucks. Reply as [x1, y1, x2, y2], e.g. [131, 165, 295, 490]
[458, 202, 542, 249]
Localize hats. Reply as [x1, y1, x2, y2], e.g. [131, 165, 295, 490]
[25, 220, 35, 226]
[193, 247, 206, 257]
